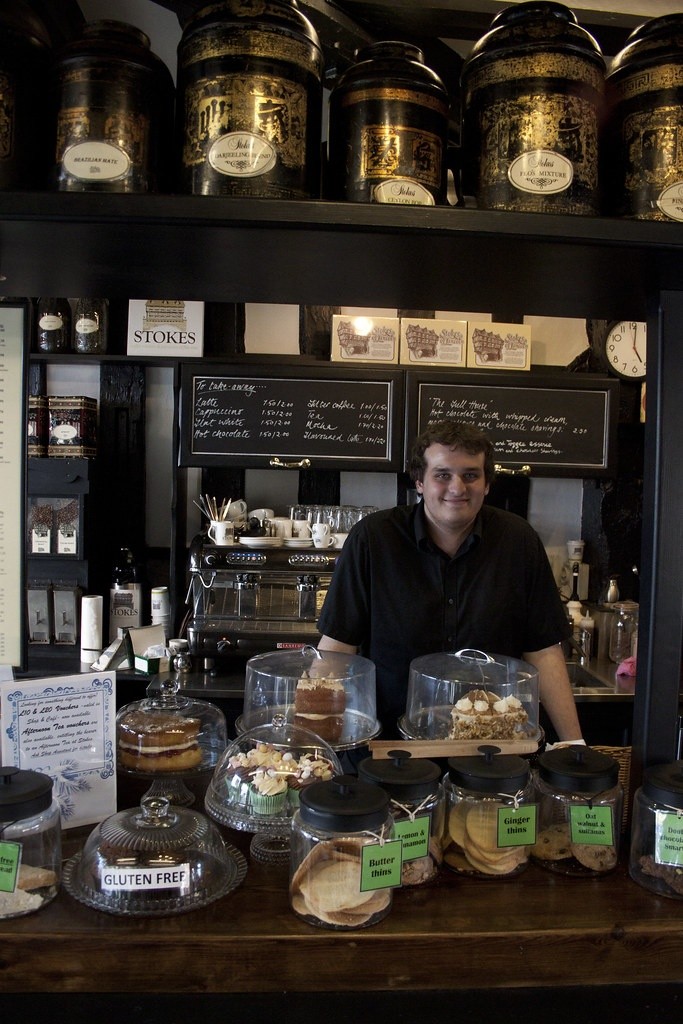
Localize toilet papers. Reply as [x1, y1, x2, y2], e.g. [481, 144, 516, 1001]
[81, 594, 103, 650]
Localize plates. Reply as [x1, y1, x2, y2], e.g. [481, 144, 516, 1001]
[239, 537, 313, 548]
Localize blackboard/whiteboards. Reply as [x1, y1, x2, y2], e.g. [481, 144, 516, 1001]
[177, 362, 405, 473]
[403, 370, 623, 478]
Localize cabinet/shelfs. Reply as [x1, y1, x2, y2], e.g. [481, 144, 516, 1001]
[0, 184, 683, 796]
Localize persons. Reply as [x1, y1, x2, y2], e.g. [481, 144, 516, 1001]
[313, 423, 584, 742]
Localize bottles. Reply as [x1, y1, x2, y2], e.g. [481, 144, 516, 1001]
[581, 608, 594, 656]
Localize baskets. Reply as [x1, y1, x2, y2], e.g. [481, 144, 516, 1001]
[539, 747, 630, 831]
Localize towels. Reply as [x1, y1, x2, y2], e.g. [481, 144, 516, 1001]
[616, 655, 636, 676]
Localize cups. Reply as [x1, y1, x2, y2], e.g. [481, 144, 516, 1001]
[150, 587, 170, 640]
[310, 523, 335, 548]
[566, 540, 585, 569]
[168, 639, 188, 656]
[208, 521, 234, 546]
[217, 498, 380, 537]
